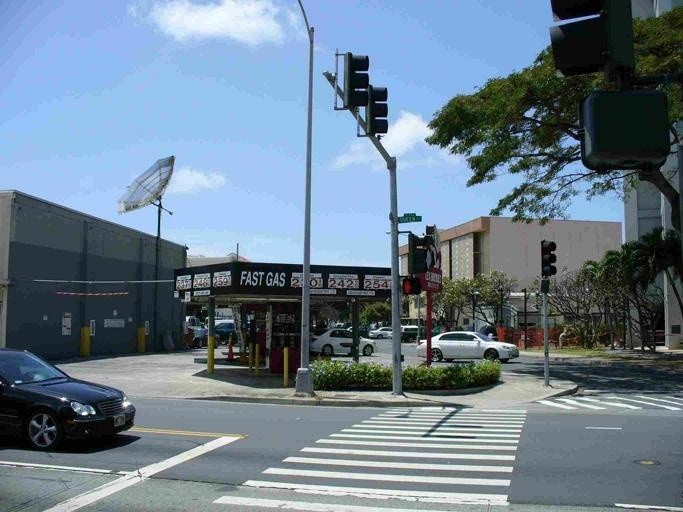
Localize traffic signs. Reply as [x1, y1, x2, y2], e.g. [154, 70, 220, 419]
[397, 211, 423, 224]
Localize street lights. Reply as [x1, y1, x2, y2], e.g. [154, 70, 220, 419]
[519, 287, 530, 352]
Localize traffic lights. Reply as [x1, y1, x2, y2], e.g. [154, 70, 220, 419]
[542, 239, 558, 278]
[544, 0, 618, 80]
[398, 276, 420, 296]
[541, 279, 549, 292]
[411, 233, 428, 276]
[346, 51, 371, 113]
[367, 83, 391, 138]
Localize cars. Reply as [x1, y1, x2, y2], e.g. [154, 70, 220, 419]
[185, 312, 418, 356]
[414, 330, 521, 364]
[0, 349, 138, 450]
[559, 329, 577, 344]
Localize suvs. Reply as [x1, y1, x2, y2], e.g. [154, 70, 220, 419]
[476, 324, 514, 343]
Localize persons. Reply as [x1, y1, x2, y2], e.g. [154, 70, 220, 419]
[560, 329, 570, 346]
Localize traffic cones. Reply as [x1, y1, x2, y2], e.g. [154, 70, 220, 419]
[225, 341, 236, 362]
